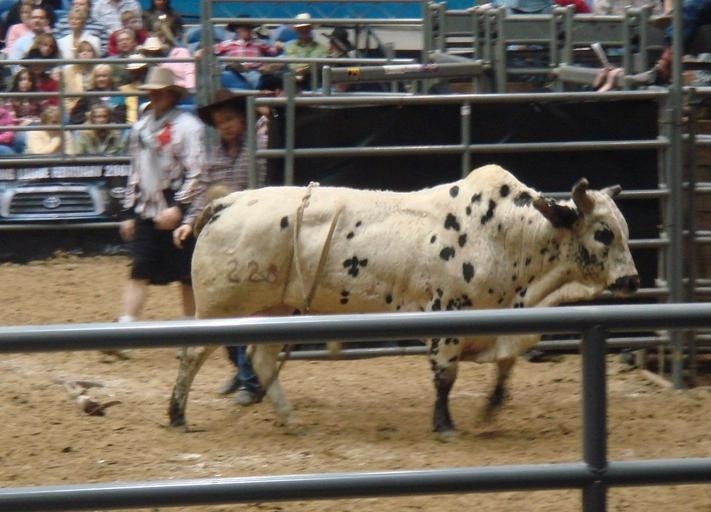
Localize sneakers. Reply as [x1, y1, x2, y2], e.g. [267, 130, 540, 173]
[219, 375, 255, 407]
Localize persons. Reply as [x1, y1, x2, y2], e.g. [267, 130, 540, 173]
[173, 91, 270, 407]
[102, 68, 208, 360]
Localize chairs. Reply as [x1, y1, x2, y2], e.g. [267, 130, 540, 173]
[0, 0, 318, 164]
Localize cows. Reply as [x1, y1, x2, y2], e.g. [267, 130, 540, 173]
[166, 162, 641, 443]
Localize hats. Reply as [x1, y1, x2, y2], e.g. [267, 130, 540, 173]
[227, 14, 261, 32]
[125, 54, 147, 70]
[322, 27, 355, 52]
[136, 36, 165, 52]
[197, 88, 245, 125]
[294, 12, 312, 28]
[138, 66, 188, 100]
